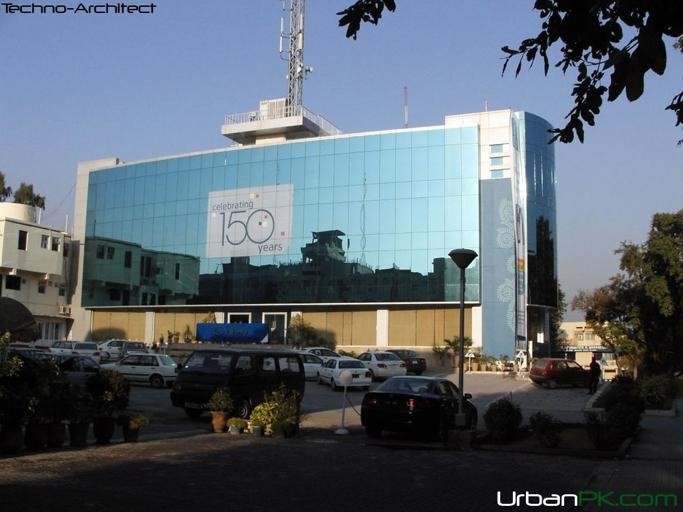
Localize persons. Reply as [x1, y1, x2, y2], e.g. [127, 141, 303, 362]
[587, 356, 602, 393]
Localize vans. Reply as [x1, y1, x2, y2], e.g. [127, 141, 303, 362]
[168, 349, 305, 418]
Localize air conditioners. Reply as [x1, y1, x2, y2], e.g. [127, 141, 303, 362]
[57, 305, 70, 315]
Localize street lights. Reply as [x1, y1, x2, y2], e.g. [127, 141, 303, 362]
[447, 247, 478, 427]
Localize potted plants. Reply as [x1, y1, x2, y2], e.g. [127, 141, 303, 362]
[250, 401, 269, 437]
[59, 379, 94, 448]
[271, 384, 303, 436]
[115, 411, 149, 442]
[434, 335, 520, 377]
[4, 353, 62, 450]
[200, 387, 234, 435]
[226, 416, 246, 435]
[84, 362, 130, 446]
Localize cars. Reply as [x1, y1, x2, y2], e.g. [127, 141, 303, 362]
[315, 358, 372, 390]
[47, 341, 79, 354]
[45, 354, 99, 382]
[272, 352, 324, 380]
[360, 375, 478, 439]
[72, 342, 98, 363]
[386, 349, 427, 373]
[303, 347, 354, 361]
[528, 358, 590, 388]
[100, 354, 177, 387]
[5, 344, 52, 367]
[98, 338, 127, 359]
[353, 351, 407, 379]
[118, 341, 149, 360]
